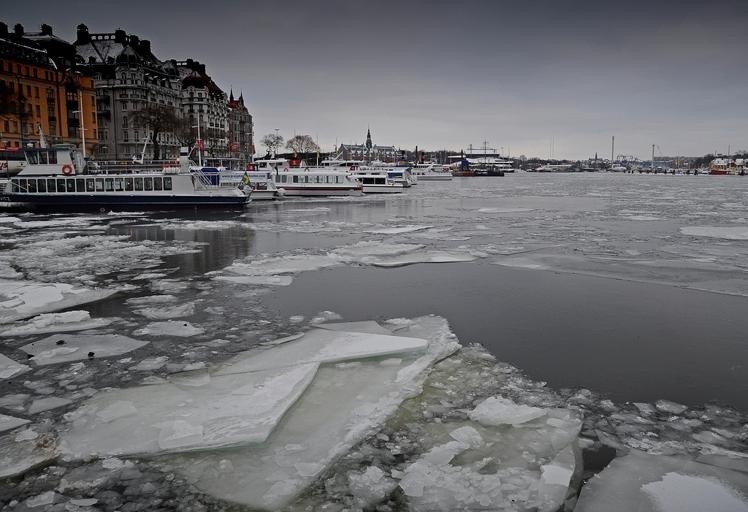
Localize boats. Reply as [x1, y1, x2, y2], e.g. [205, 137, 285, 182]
[0, 89, 514, 210]
[607, 164, 627, 172]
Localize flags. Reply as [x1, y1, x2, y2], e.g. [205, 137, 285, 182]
[241, 171, 251, 185]
